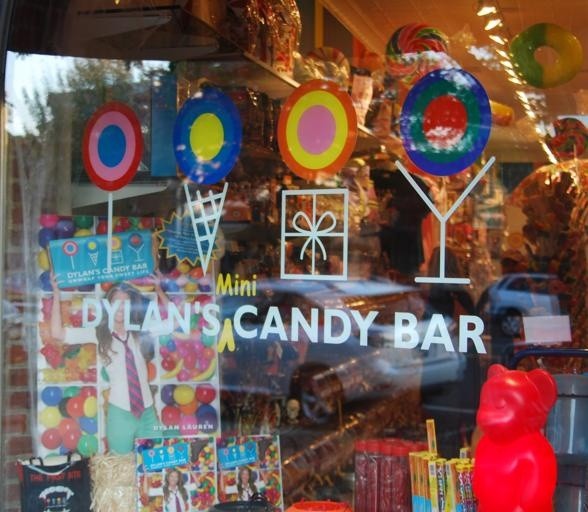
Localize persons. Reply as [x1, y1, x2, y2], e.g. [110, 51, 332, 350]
[238, 467, 256, 501]
[96, 270, 175, 455]
[163, 468, 189, 512]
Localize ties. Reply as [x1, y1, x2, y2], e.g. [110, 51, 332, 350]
[171, 490, 181, 512]
[110, 330, 145, 419]
[245, 488, 253, 500]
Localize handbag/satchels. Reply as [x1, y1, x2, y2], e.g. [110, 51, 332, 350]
[17, 450, 93, 512]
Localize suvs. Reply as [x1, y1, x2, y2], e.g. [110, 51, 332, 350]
[481, 271, 571, 337]
[132, 276, 465, 426]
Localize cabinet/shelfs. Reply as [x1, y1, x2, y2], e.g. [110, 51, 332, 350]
[56, 0, 464, 511]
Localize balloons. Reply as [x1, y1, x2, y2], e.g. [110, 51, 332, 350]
[155, 260, 220, 432]
[256, 438, 281, 507]
[189, 438, 216, 512]
[216, 438, 238, 503]
[38, 214, 131, 290]
[134, 439, 163, 511]
[38, 339, 98, 457]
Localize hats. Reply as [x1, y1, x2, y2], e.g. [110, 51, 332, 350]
[501, 249, 524, 262]
